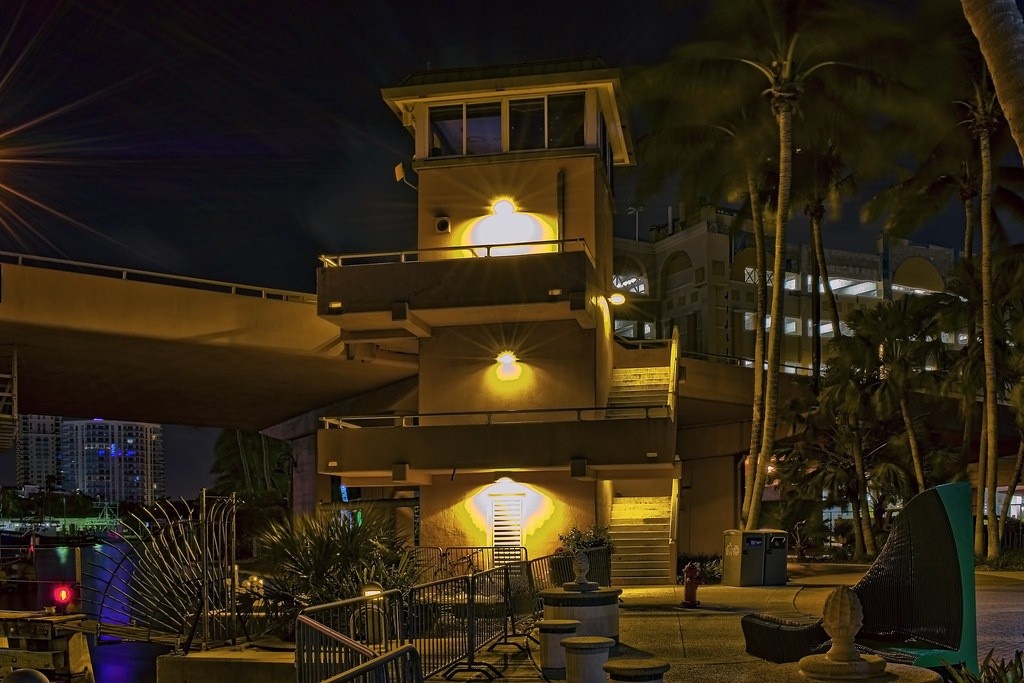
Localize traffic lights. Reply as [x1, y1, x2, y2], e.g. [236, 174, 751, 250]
[55, 587, 72, 603]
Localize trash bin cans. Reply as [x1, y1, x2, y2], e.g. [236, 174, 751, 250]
[758, 528, 789, 586]
[721, 529, 765, 586]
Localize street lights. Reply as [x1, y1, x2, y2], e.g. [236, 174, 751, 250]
[626, 205, 650, 243]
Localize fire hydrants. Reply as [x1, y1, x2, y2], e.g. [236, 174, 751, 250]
[681, 561, 703, 609]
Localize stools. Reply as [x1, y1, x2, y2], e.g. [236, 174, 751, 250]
[533, 620, 582, 680]
[602, 658, 671, 683]
[559, 636, 615, 683]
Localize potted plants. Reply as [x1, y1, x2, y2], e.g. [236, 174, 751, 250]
[548, 525, 619, 589]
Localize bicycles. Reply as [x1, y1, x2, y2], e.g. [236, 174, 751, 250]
[432, 549, 495, 598]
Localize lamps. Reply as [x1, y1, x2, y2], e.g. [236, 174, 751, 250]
[274, 452, 297, 477]
[394, 162, 418, 192]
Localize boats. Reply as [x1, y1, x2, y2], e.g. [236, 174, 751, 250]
[0, 494, 127, 683]
